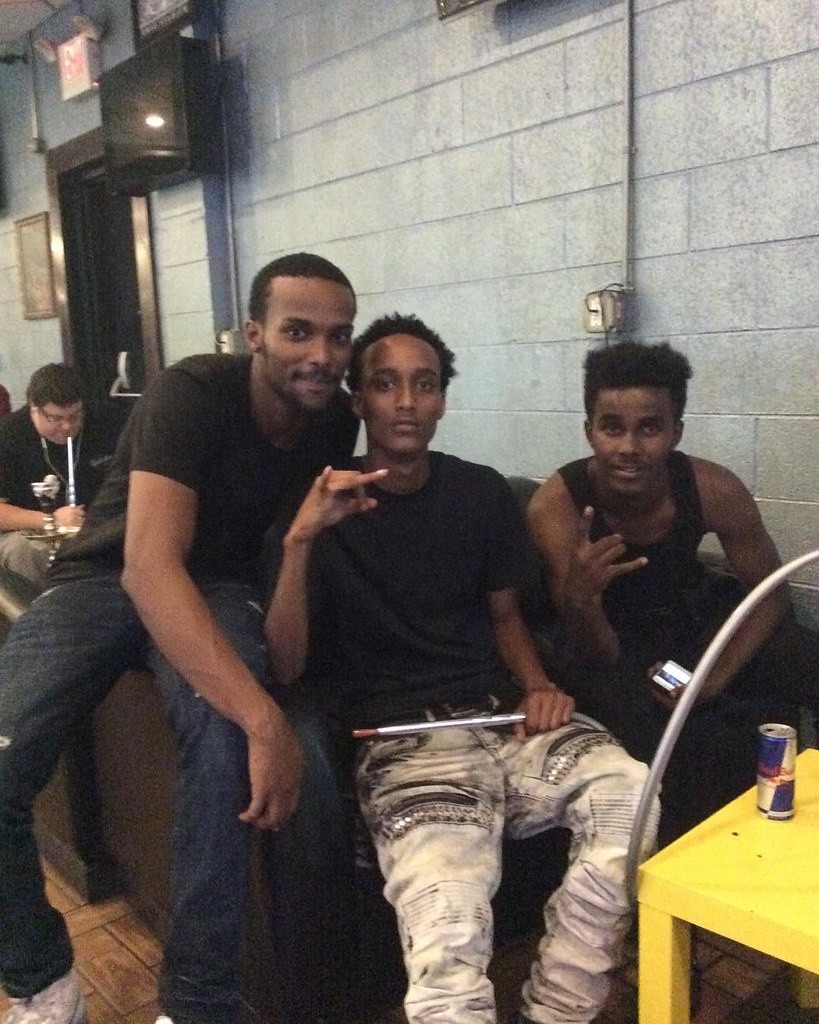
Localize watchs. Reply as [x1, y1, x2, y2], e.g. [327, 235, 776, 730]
[44, 512, 56, 537]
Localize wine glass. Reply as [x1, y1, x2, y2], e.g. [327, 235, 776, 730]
[30, 482, 60, 535]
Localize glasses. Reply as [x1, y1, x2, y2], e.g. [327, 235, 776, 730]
[35, 402, 86, 424]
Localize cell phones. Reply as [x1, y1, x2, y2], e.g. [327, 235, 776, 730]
[650, 659, 694, 695]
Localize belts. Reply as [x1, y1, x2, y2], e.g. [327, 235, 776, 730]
[373, 684, 523, 727]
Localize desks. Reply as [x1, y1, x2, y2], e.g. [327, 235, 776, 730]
[635, 747, 819, 1024]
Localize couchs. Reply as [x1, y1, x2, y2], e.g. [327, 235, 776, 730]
[91, 476, 801, 1024]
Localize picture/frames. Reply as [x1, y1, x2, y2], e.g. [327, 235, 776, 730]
[14, 212, 57, 318]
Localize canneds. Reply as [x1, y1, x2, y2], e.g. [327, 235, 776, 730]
[756, 722, 796, 819]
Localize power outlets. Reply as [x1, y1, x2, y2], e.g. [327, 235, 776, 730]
[583, 292, 628, 332]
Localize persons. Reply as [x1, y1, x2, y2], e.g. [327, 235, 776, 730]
[262, 310, 664, 1024]
[0, 251, 360, 1023]
[0, 363, 118, 593]
[522, 341, 809, 853]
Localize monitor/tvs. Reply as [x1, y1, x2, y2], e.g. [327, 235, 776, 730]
[99, 32, 218, 198]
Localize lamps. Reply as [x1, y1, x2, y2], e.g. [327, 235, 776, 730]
[31, 15, 106, 103]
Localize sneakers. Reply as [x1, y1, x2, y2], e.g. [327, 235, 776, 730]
[5, 967, 88, 1024]
